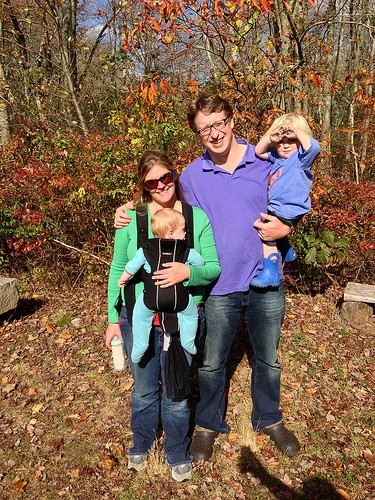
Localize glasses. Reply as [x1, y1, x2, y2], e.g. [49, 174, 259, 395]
[197, 111, 231, 136]
[144, 172, 174, 191]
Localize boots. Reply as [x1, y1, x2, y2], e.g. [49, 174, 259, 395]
[250, 252, 280, 289]
[276, 239, 296, 262]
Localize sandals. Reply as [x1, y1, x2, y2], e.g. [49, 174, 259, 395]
[262, 421, 300, 457]
[189, 429, 217, 462]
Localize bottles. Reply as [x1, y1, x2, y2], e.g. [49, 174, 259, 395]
[110, 335, 127, 371]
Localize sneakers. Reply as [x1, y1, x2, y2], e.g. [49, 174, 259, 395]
[128, 455, 149, 471]
[171, 463, 193, 483]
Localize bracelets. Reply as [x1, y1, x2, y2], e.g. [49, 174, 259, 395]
[289, 224, 295, 236]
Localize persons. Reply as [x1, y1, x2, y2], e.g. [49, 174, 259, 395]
[250, 113, 320, 287]
[105, 150, 221, 482]
[118, 207, 205, 363]
[113, 93, 300, 461]
